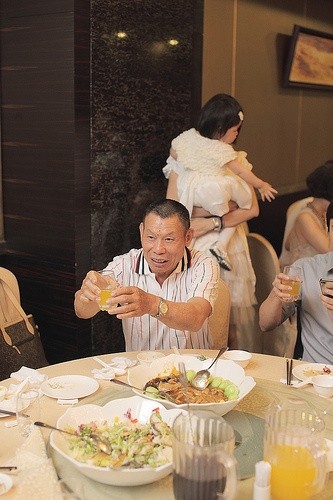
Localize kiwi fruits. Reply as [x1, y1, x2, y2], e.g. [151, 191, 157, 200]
[144, 386, 161, 398]
[186, 370, 239, 401]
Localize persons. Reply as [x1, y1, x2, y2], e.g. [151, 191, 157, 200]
[74, 199, 220, 352]
[163, 123, 260, 352]
[259, 200, 333, 364]
[170, 93, 278, 270]
[280, 160, 333, 270]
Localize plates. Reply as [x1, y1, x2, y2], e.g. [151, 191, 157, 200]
[0, 473, 13, 495]
[291, 363, 333, 384]
[0, 391, 31, 418]
[40, 375, 99, 400]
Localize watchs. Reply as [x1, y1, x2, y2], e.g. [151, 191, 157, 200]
[212, 217, 221, 231]
[151, 297, 168, 318]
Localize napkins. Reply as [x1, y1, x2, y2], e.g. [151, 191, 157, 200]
[7, 378, 27, 396]
[0, 427, 78, 500]
[92, 367, 115, 381]
[9, 364, 49, 387]
[111, 356, 137, 370]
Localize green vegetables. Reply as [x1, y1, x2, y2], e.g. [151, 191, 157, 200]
[67, 423, 177, 470]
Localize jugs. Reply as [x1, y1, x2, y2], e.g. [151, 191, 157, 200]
[264, 396, 328, 500]
[172, 408, 239, 500]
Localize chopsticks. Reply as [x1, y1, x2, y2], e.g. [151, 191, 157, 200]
[0, 409, 30, 418]
[286, 359, 293, 385]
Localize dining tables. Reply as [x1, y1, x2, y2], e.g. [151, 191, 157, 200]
[0, 349, 333, 500]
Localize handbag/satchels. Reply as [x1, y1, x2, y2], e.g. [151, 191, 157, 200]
[0, 278, 49, 381]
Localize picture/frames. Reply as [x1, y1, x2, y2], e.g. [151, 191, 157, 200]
[280, 24, 333, 93]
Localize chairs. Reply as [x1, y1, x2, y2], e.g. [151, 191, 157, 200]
[247, 231, 292, 359]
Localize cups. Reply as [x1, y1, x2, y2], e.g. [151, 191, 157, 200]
[281, 266, 301, 303]
[94, 269, 118, 311]
[16, 390, 41, 438]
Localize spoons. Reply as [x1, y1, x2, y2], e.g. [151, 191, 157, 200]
[191, 346, 229, 391]
[93, 356, 126, 376]
[34, 421, 112, 454]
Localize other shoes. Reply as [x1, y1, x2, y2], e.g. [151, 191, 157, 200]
[209, 246, 232, 271]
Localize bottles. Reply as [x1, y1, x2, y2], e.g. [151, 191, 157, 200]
[253, 459, 272, 500]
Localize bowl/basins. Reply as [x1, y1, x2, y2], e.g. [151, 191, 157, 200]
[49, 395, 189, 487]
[223, 350, 254, 368]
[311, 375, 333, 396]
[128, 353, 257, 417]
[136, 351, 166, 366]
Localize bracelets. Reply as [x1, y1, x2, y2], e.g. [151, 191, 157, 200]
[220, 217, 225, 228]
